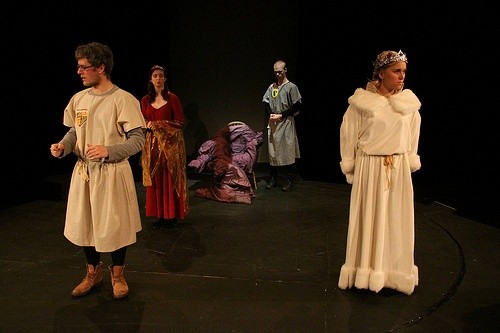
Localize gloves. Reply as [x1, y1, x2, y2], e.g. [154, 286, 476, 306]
[345, 172, 354, 184]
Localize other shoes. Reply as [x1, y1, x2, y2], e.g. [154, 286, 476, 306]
[266, 180, 278, 188]
[282, 178, 293, 189]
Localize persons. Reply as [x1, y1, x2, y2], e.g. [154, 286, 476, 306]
[51, 42, 146, 298]
[262, 61, 302, 190]
[141, 66, 190, 223]
[338, 51, 422, 295]
[188, 125, 264, 173]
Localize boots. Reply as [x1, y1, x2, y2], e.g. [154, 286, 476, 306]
[108, 264, 130, 298]
[72, 261, 103, 295]
